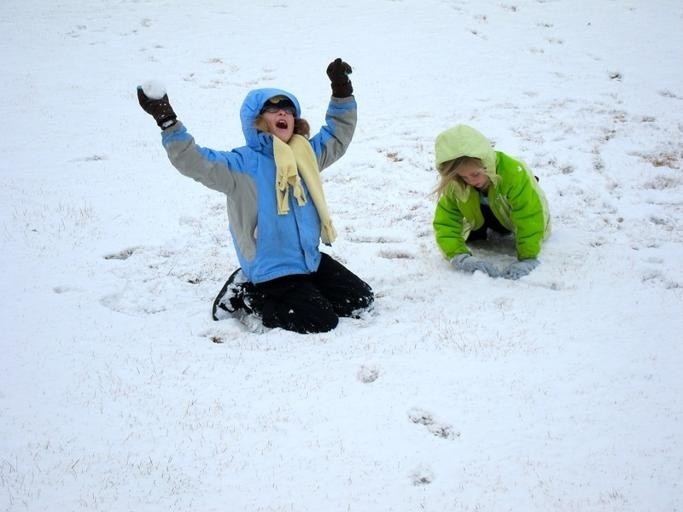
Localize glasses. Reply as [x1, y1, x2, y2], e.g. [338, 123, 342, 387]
[262, 106, 294, 114]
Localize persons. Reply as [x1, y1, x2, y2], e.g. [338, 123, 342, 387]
[433, 125, 550, 280]
[137, 58, 374, 334]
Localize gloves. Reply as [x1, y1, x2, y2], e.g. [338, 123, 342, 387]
[136, 85, 176, 130]
[453, 253, 540, 279]
[326, 58, 353, 97]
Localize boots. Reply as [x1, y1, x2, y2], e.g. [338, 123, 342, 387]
[212, 268, 251, 321]
